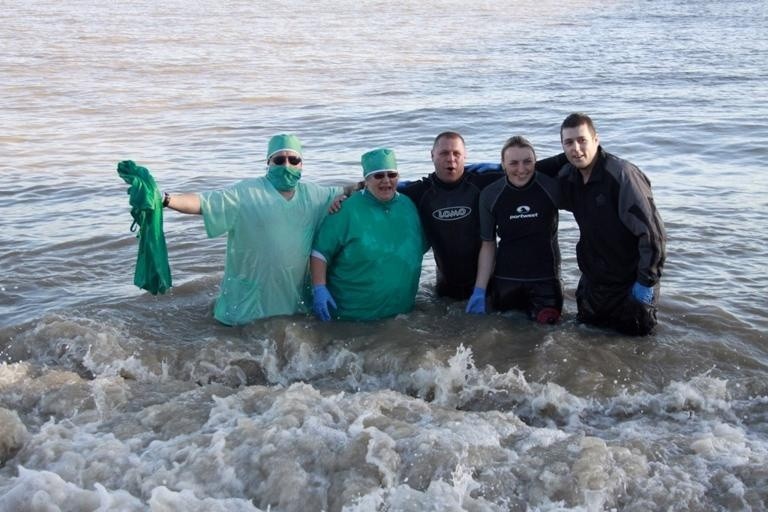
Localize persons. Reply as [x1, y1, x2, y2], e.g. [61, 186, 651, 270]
[161, 133, 411, 326]
[465, 112, 667, 326]
[328, 131, 496, 301]
[465, 135, 575, 324]
[310, 148, 431, 322]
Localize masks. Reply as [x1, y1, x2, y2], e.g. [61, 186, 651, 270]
[265, 165, 303, 192]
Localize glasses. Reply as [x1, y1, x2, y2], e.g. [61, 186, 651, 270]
[269, 156, 303, 166]
[371, 173, 398, 180]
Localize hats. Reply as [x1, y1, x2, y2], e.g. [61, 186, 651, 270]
[266, 134, 306, 165]
[360, 148, 398, 179]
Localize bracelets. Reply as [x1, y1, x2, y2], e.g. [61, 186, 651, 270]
[163, 192, 171, 207]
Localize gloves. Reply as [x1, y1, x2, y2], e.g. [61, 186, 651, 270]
[464, 163, 501, 175]
[311, 285, 338, 323]
[465, 288, 487, 316]
[631, 282, 654, 307]
